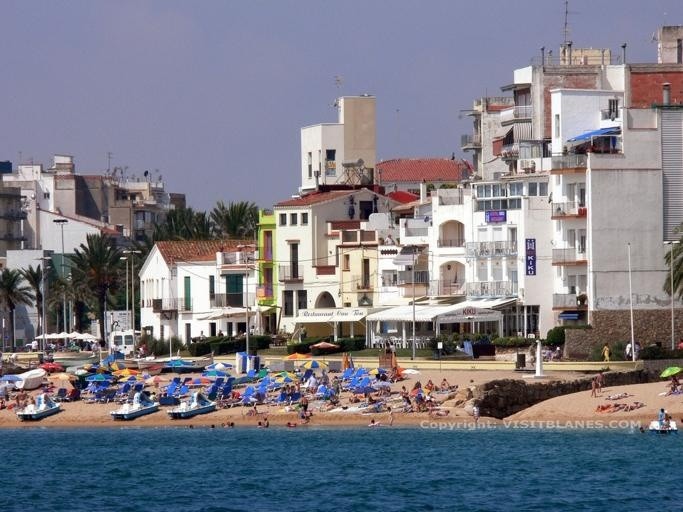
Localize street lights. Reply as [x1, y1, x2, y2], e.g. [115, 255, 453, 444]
[118, 249, 142, 359]
[32, 219, 68, 351]
[663, 240, 681, 350]
[238, 244, 257, 356]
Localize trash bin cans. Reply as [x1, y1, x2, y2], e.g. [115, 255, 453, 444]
[517, 354, 525, 366]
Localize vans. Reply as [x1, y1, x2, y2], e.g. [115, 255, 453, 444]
[108, 330, 136, 358]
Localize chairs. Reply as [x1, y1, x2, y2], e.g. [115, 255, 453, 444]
[52, 366, 404, 406]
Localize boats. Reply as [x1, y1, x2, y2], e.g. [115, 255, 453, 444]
[116, 358, 214, 373]
[167, 401, 217, 419]
[111, 401, 160, 420]
[18, 403, 61, 420]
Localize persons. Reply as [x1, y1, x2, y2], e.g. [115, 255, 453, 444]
[0, 322, 683, 434]
